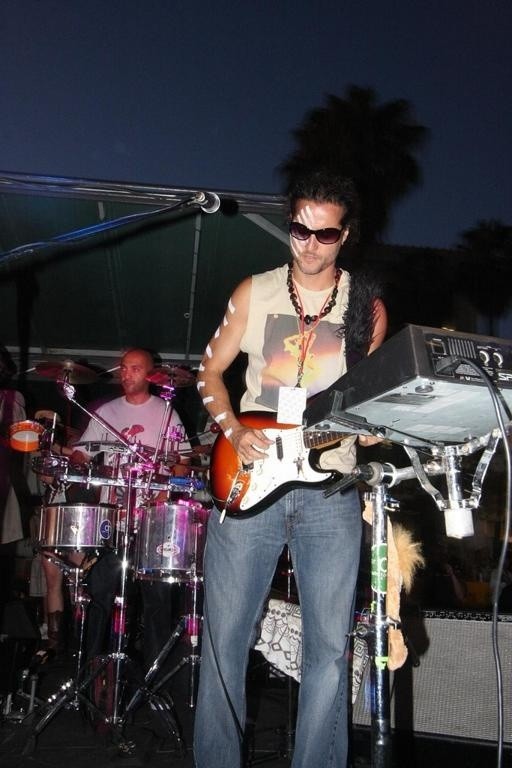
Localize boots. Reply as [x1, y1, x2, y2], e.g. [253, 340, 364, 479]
[45, 609, 64, 654]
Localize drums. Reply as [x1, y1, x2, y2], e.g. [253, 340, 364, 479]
[36, 502, 119, 554]
[6, 419, 48, 454]
[31, 456, 90, 481]
[128, 500, 210, 586]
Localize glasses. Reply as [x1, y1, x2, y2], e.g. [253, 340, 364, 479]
[288, 221, 344, 245]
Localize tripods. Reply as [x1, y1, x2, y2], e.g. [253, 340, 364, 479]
[114, 574, 205, 742]
[13, 463, 188, 765]
[1, 556, 97, 734]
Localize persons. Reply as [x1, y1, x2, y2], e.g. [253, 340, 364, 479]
[0, 346, 192, 662]
[191, 176, 390, 768]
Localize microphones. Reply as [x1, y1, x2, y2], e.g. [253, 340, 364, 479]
[197, 188, 240, 219]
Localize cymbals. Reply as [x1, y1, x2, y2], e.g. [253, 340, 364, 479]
[35, 360, 99, 385]
[138, 360, 201, 393]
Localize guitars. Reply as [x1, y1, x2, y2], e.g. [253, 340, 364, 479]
[209, 411, 359, 520]
